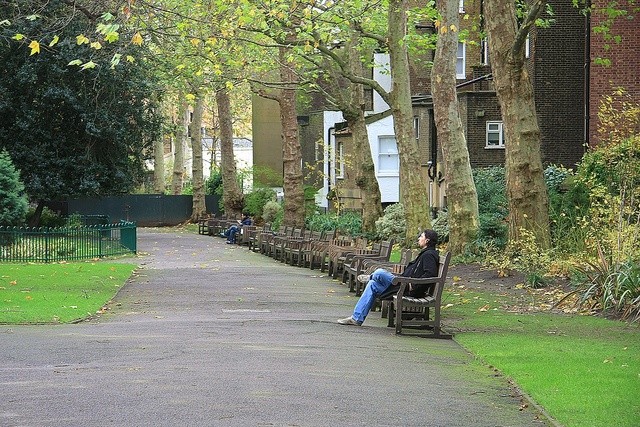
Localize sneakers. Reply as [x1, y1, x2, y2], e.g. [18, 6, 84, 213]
[338, 316, 361, 326]
[357, 275, 372, 284]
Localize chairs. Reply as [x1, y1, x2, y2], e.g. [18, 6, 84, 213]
[197, 205, 393, 296]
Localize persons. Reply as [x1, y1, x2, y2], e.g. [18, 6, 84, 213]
[337, 229, 440, 326]
[220, 212, 251, 244]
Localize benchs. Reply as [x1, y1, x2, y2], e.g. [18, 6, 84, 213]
[387, 250, 452, 336]
